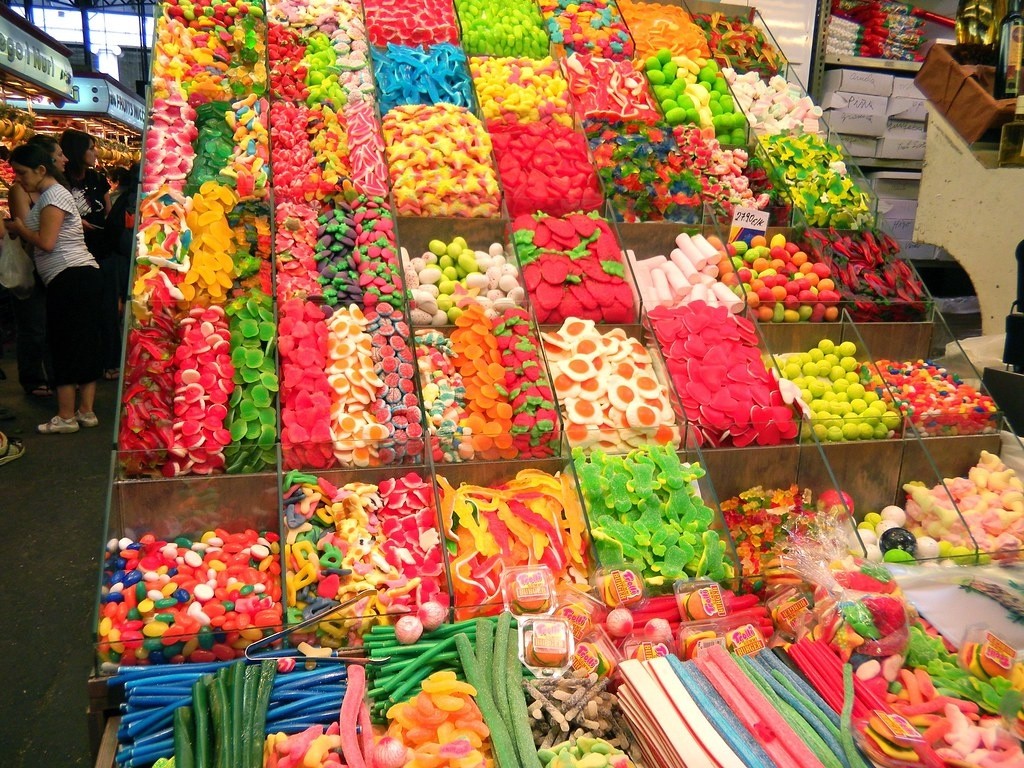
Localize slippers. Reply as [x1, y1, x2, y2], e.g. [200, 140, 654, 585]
[32, 385, 53, 396]
[105, 369, 121, 380]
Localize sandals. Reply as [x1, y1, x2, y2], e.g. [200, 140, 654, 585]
[0, 441, 25, 467]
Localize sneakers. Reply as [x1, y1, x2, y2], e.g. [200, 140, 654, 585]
[38, 415, 80, 434]
[75, 411, 98, 427]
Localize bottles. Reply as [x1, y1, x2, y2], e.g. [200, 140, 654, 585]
[993, 0, 1024, 100]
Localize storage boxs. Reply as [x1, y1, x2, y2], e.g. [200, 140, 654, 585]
[876, 120, 925, 141]
[828, 134, 879, 158]
[876, 136, 926, 160]
[821, 91, 889, 117]
[88, 0, 1024, 679]
[871, 178, 920, 200]
[877, 220, 915, 240]
[890, 77, 929, 100]
[913, 43, 1018, 146]
[877, 199, 919, 220]
[824, 68, 894, 98]
[887, 98, 926, 122]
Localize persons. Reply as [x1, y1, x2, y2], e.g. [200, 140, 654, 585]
[0, 129, 141, 433]
[0, 430, 26, 468]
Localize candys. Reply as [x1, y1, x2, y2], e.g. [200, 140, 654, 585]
[97, 0, 1024, 768]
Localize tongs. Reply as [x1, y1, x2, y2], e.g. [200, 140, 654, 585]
[246, 585, 392, 665]
